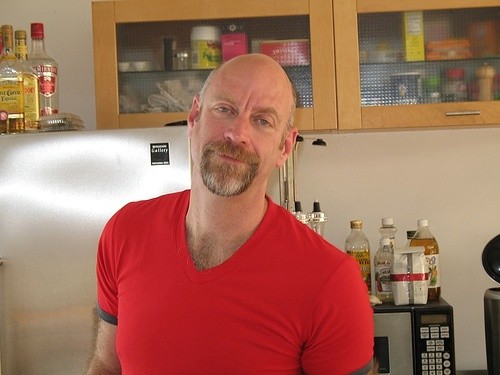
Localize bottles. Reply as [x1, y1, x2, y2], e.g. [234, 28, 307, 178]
[346, 220, 372, 298]
[374, 236, 395, 302]
[426, 63, 496, 103]
[164, 25, 222, 70]
[378, 217, 396, 253]
[394, 246, 428, 306]
[296, 200, 326, 237]
[409, 218, 441, 301]
[406, 231, 418, 246]
[0, 22, 59, 135]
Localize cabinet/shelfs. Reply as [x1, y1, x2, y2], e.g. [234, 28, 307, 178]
[91, 0, 500, 130]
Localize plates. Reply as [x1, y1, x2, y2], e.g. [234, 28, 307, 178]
[117, 59, 155, 72]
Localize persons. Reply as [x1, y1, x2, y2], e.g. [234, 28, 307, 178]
[87, 53, 374, 375]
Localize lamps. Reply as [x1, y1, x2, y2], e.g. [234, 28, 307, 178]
[280, 135, 327, 203]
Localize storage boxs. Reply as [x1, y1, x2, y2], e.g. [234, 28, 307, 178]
[251, 40, 310, 66]
[221, 24, 248, 63]
[401, 10, 424, 61]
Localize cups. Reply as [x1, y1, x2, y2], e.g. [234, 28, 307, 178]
[390, 72, 425, 105]
